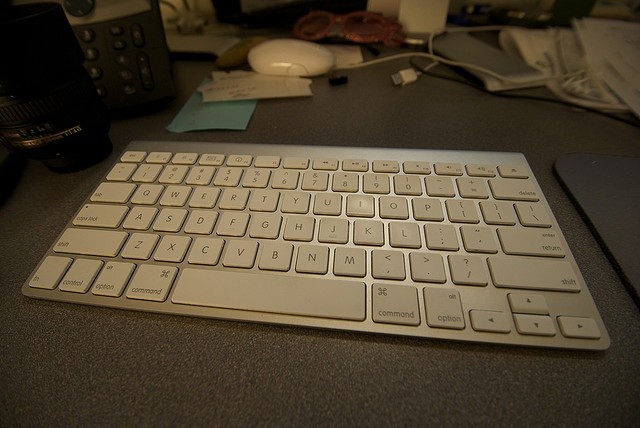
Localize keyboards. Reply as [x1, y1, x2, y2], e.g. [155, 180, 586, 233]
[22, 140, 614, 352]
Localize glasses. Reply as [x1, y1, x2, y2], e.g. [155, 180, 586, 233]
[293, 11, 395, 58]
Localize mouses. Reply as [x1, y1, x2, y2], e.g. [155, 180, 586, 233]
[248, 39, 336, 78]
